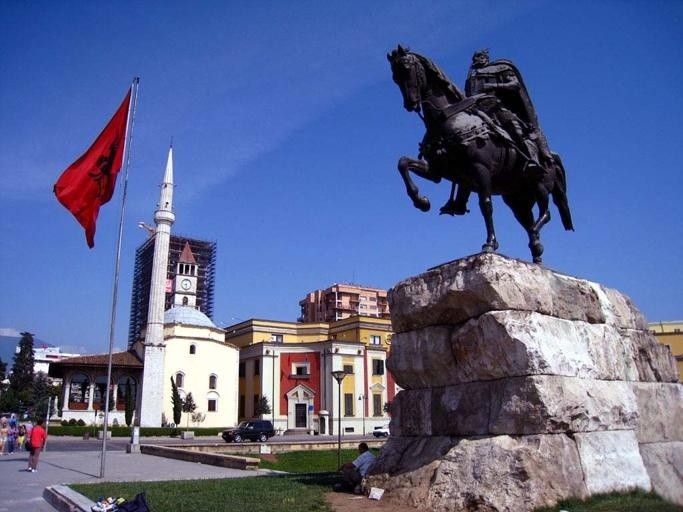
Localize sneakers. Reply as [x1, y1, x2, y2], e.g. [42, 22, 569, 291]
[27, 467, 38, 473]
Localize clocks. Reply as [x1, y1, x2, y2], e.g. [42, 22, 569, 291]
[181, 279, 191, 290]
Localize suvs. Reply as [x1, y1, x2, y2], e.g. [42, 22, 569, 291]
[222, 420, 274, 444]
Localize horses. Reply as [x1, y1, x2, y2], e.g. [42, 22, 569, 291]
[387, 44, 575, 267]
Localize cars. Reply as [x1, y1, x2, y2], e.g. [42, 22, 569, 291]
[373, 424, 390, 437]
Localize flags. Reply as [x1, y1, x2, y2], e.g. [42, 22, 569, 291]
[52, 86, 132, 250]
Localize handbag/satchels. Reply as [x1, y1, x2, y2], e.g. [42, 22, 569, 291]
[25, 437, 31, 451]
[116, 493, 149, 511]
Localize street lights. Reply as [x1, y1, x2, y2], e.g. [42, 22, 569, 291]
[330, 370, 350, 471]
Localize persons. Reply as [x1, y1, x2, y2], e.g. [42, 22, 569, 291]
[465, 46, 554, 171]
[333, 442, 377, 489]
[0, 413, 47, 473]
